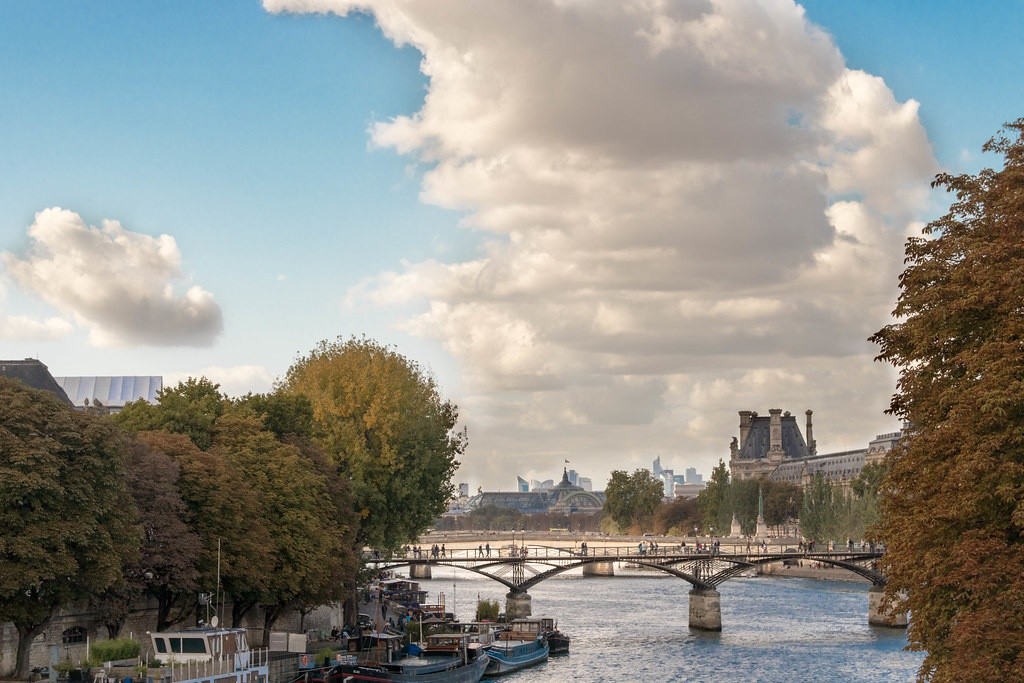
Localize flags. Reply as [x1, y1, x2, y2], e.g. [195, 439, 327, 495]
[565, 459, 569, 463]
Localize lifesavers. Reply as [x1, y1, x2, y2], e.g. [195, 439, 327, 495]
[337, 654, 341, 661]
[303, 656, 307, 665]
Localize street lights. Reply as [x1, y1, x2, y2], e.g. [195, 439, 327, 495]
[520, 525, 525, 548]
[511, 526, 515, 545]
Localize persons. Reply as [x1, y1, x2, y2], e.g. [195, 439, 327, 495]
[479, 544, 484, 557]
[331, 543, 447, 642]
[486, 542, 489, 557]
[581, 536, 873, 556]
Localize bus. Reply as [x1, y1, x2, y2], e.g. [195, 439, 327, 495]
[550, 528, 569, 532]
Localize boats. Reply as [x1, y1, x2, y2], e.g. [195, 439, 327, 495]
[366, 570, 460, 624]
[548, 619, 571, 656]
[342, 612, 490, 683]
[89, 538, 309, 683]
[482, 605, 549, 674]
